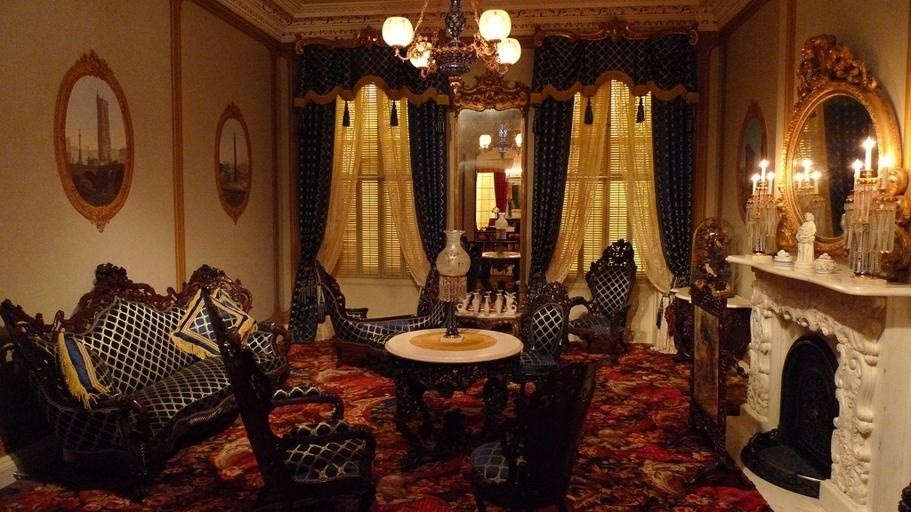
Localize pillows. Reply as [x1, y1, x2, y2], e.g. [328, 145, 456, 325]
[56, 291, 252, 404]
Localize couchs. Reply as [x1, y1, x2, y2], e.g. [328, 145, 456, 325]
[1, 265, 290, 499]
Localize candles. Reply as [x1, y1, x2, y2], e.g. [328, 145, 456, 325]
[745, 159, 780, 192]
[795, 159, 822, 192]
[851, 139, 893, 187]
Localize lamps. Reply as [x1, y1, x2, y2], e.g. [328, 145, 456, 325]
[433, 229, 469, 343]
[378, 1, 524, 80]
[477, 116, 520, 157]
[494, 212, 508, 254]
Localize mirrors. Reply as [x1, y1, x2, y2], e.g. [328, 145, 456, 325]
[451, 72, 526, 293]
[791, 91, 881, 242]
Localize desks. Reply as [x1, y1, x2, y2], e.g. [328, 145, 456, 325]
[384, 326, 524, 431]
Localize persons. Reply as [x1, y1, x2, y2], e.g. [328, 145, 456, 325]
[795, 210, 818, 264]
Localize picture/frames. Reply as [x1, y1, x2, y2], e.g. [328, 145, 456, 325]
[55, 50, 256, 232]
[733, 99, 766, 225]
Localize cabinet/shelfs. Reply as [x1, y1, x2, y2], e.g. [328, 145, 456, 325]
[665, 287, 752, 374]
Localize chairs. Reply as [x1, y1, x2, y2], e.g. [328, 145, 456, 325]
[201, 288, 380, 512]
[499, 279, 572, 414]
[465, 356, 599, 510]
[313, 254, 401, 372]
[566, 238, 642, 360]
